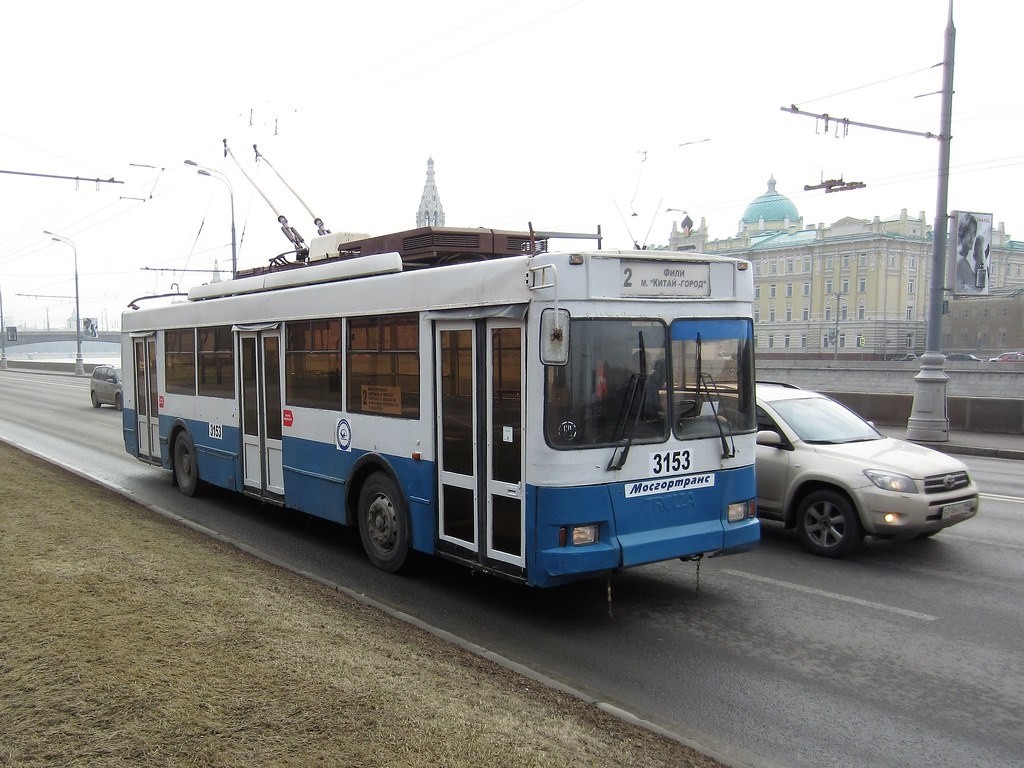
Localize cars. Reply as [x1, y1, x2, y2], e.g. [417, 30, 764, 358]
[946, 352, 981, 361]
[989, 350, 1018, 361]
[1002, 355, 1024, 362]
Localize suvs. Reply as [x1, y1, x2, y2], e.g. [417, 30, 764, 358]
[661, 381, 980, 556]
[90, 362, 123, 411]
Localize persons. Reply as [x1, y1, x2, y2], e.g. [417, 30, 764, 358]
[956, 213, 986, 294]
[84, 318, 97, 337]
[625, 352, 666, 434]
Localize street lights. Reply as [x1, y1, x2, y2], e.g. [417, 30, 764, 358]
[184, 159, 237, 277]
[44, 230, 86, 374]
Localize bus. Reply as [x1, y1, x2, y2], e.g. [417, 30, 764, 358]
[120, 223, 763, 586]
